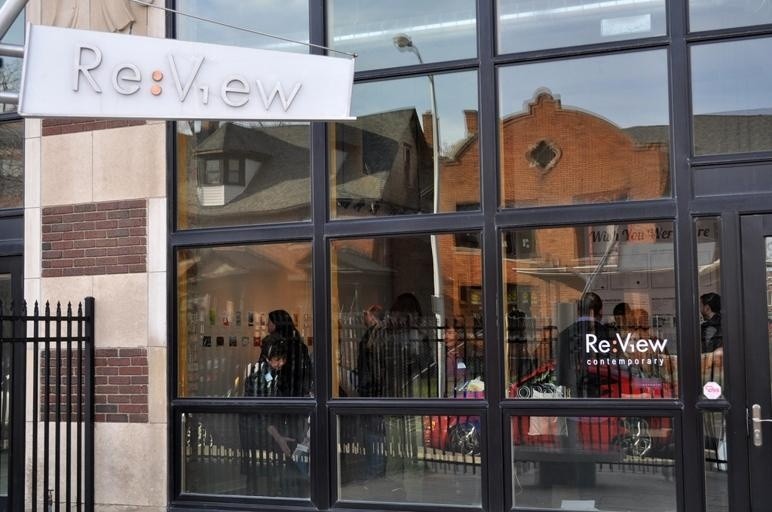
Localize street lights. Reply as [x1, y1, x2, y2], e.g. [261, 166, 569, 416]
[393, 35, 445, 391]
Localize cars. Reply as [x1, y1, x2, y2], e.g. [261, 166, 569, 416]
[422, 362, 674, 457]
[183, 371, 399, 453]
